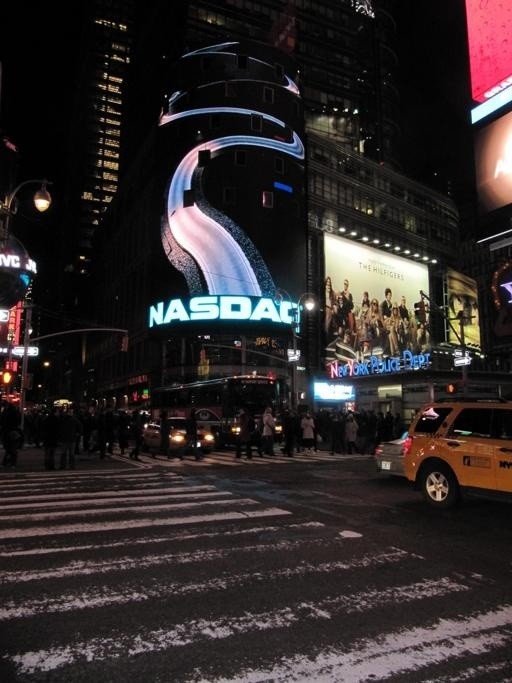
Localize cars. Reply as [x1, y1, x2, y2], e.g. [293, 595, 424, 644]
[142, 415, 218, 455]
[373, 431, 412, 482]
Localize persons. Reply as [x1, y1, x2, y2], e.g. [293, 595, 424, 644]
[324, 274, 430, 358]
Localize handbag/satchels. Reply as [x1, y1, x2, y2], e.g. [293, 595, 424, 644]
[272, 427, 277, 443]
[316, 431, 323, 443]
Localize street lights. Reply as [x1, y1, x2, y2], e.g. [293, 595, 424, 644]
[3, 320, 33, 403]
[269, 285, 318, 411]
[1, 177, 57, 241]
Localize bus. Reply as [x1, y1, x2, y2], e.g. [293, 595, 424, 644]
[147, 371, 286, 444]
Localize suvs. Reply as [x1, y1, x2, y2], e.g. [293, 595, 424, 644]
[400, 400, 512, 513]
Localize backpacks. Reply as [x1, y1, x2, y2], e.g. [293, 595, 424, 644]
[247, 414, 255, 433]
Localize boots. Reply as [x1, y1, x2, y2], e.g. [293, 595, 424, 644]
[304, 448, 309, 456]
[310, 447, 315, 456]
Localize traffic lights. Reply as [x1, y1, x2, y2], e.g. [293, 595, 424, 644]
[3, 370, 13, 384]
[122, 337, 128, 350]
[415, 299, 426, 326]
[200, 349, 206, 361]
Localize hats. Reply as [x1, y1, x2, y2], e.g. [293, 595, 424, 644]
[346, 414, 354, 420]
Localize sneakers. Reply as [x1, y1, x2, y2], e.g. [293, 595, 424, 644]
[329, 448, 376, 457]
[7, 447, 143, 470]
[152, 450, 297, 459]
